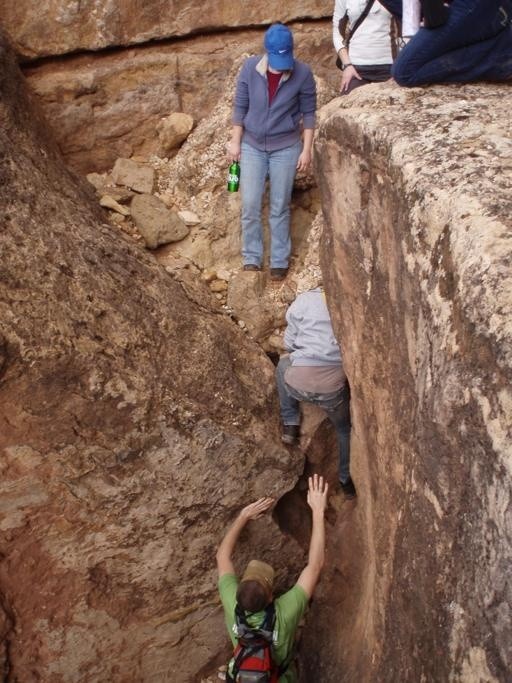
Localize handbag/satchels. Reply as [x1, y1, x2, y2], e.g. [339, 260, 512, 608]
[336, 58, 345, 71]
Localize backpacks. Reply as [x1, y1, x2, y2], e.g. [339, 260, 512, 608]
[225, 601, 298, 683]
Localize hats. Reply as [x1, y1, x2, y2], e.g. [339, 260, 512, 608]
[262, 22, 296, 72]
[238, 559, 275, 604]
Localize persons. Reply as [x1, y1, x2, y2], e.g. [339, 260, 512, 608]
[273, 268, 355, 502]
[329, 1, 512, 88]
[214, 474, 329, 683]
[228, 21, 317, 282]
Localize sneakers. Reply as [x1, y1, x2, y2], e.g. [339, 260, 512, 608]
[281, 424, 300, 447]
[339, 476, 357, 501]
[269, 265, 290, 281]
[243, 263, 260, 272]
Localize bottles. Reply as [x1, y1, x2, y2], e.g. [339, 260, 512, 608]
[227, 161, 241, 193]
